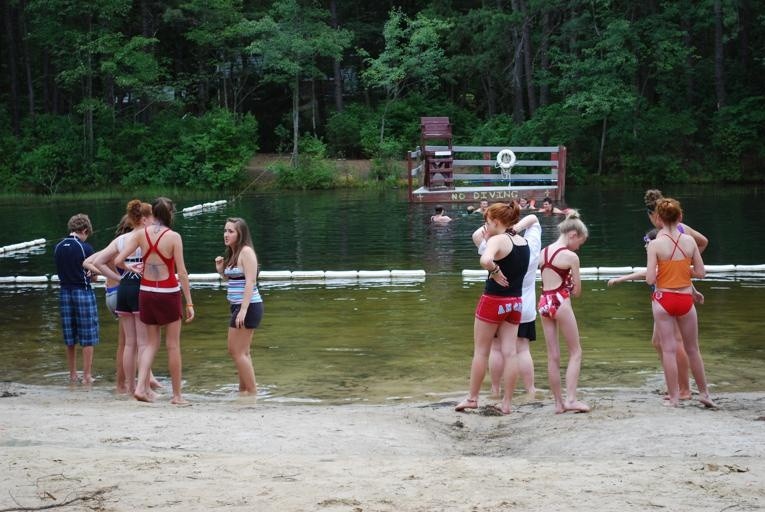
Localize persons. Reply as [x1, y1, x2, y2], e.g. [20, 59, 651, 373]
[461, 196, 564, 221]
[429, 205, 452, 226]
[54, 199, 194, 405]
[606, 189, 722, 410]
[454, 202, 590, 414]
[214, 216, 264, 397]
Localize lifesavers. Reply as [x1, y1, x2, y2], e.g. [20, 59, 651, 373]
[496, 148, 516, 170]
